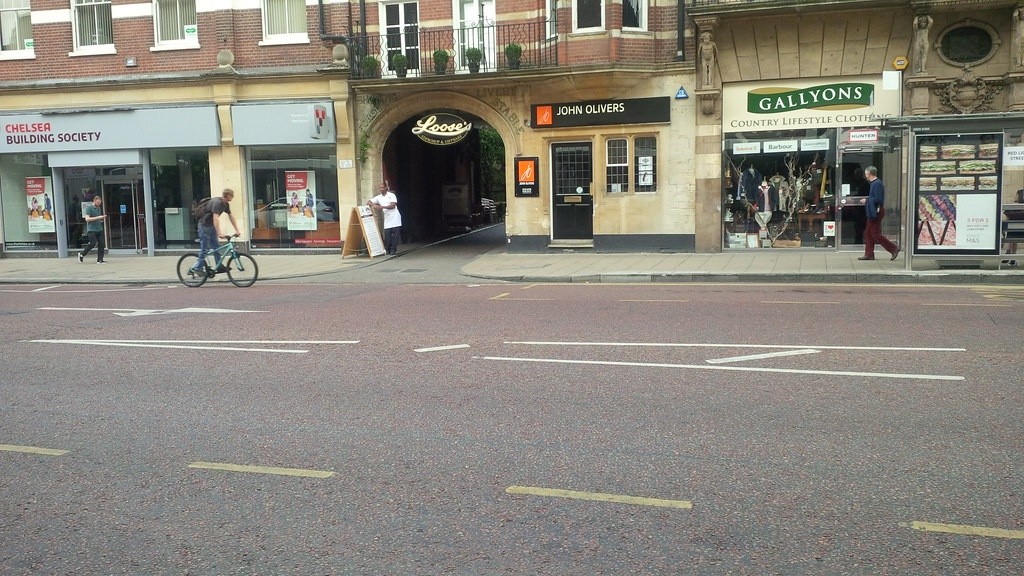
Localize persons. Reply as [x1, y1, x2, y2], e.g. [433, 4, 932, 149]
[857, 166, 901, 262]
[78, 195, 107, 265]
[1012, 5, 1024, 68]
[697, 31, 720, 88]
[1000, 188, 1024, 268]
[496, 201, 505, 223]
[290, 188, 315, 217]
[189, 189, 241, 278]
[367, 180, 404, 255]
[911, 15, 934, 74]
[30, 193, 53, 220]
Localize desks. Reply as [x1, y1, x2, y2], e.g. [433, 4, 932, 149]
[798, 211, 830, 241]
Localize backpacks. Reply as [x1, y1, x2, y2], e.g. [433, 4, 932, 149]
[193, 197, 211, 222]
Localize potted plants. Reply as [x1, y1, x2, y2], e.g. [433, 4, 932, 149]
[393, 54, 407, 77]
[466, 48, 482, 73]
[506, 45, 522, 70]
[361, 57, 382, 79]
[433, 50, 448, 75]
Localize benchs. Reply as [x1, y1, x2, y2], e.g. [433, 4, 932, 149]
[1002, 204, 1024, 243]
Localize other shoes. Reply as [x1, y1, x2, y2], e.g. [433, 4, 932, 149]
[194, 268, 204, 277]
[96, 260, 107, 265]
[858, 255, 874, 260]
[386, 250, 391, 255]
[891, 248, 901, 261]
[215, 265, 232, 272]
[78, 252, 83, 263]
[390, 250, 395, 254]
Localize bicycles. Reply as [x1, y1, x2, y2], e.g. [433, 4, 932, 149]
[176, 233, 259, 288]
